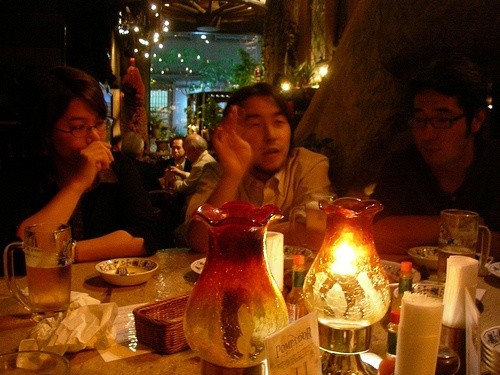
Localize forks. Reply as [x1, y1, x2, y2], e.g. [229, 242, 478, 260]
[115, 266, 129, 276]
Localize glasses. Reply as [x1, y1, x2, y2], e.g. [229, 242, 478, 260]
[405, 111, 470, 132]
[56, 116, 115, 137]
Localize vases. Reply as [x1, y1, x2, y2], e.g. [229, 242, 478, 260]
[185, 200, 289, 369]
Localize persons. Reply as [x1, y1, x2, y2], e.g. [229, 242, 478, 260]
[371, 68, 500, 261]
[111, 131, 217, 216]
[0, 65, 189, 277]
[178, 84, 331, 255]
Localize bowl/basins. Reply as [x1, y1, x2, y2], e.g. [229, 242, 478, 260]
[94, 257, 159, 286]
[381, 259, 421, 289]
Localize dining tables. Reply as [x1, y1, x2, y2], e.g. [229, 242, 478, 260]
[0, 251, 500, 375]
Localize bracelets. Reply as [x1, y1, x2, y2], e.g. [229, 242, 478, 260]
[70, 240, 76, 261]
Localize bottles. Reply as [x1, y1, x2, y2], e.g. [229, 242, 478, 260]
[378, 309, 400, 375]
[286, 255, 308, 324]
[385, 261, 414, 359]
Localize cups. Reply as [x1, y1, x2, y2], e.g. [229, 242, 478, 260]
[0, 350, 71, 375]
[436, 208, 491, 284]
[440, 324, 465, 375]
[4, 222, 74, 322]
[288, 193, 334, 256]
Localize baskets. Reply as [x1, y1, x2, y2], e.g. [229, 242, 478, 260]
[132, 294, 191, 355]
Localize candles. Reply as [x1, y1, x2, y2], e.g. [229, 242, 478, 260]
[301, 193, 394, 324]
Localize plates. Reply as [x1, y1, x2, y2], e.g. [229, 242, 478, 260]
[190, 257, 206, 274]
[488, 262, 500, 278]
[480, 326, 500, 375]
[408, 245, 438, 270]
[284, 244, 313, 260]
